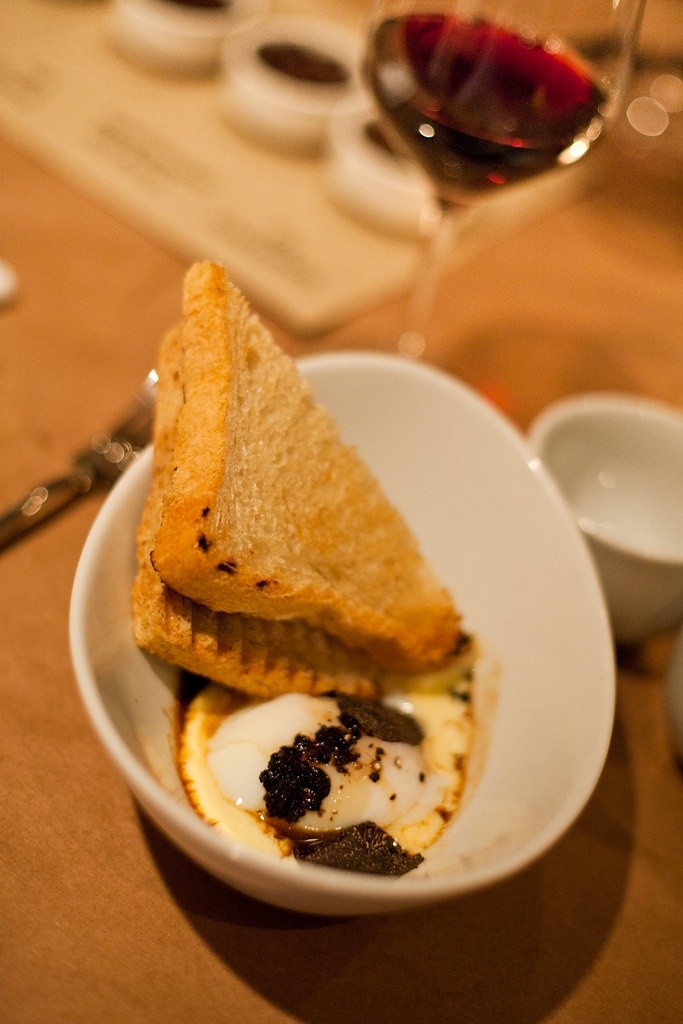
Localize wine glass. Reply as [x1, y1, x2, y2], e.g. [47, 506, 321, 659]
[360, 0, 644, 364]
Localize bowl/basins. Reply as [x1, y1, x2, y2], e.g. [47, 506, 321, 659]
[112, 1, 262, 67]
[224, 14, 362, 143]
[68, 349, 617, 917]
[326, 91, 443, 241]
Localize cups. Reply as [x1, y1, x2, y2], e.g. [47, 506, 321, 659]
[524, 389, 682, 644]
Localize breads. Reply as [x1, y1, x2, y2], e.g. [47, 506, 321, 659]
[135, 261, 474, 702]
